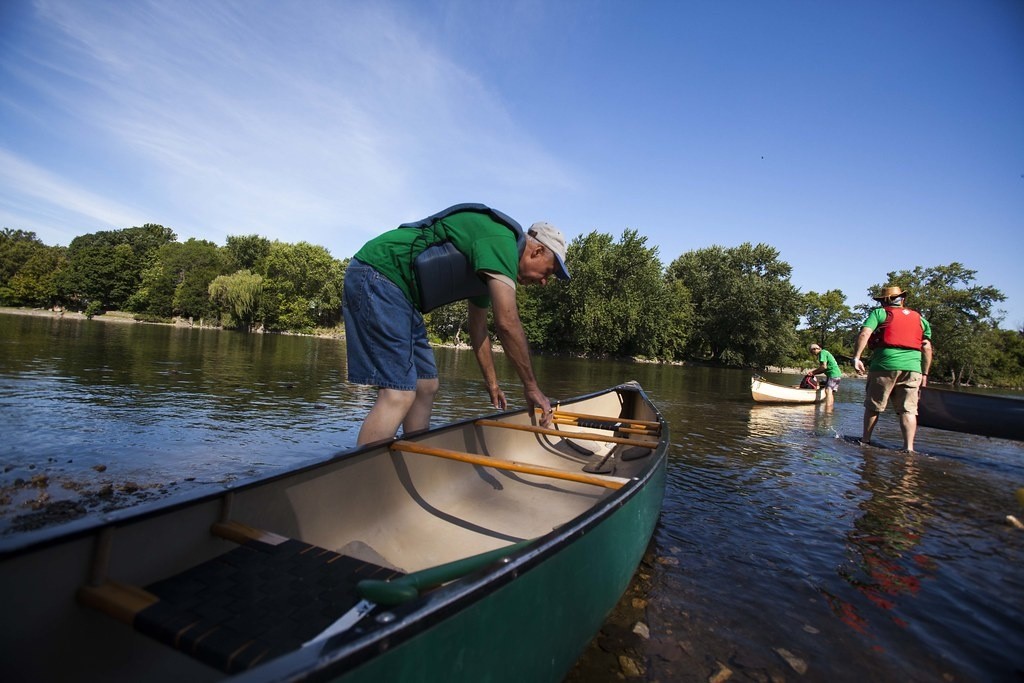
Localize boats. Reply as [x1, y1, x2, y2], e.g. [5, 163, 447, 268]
[0, 381, 675, 683]
[749, 372, 826, 403]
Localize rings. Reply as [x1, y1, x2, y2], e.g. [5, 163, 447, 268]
[549, 410, 552, 413]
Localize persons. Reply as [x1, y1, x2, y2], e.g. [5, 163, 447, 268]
[852, 287, 932, 451]
[808, 344, 842, 406]
[340, 202, 570, 448]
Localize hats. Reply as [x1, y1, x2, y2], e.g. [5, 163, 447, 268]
[809, 344, 820, 352]
[873, 286, 909, 302]
[527, 221, 571, 281]
